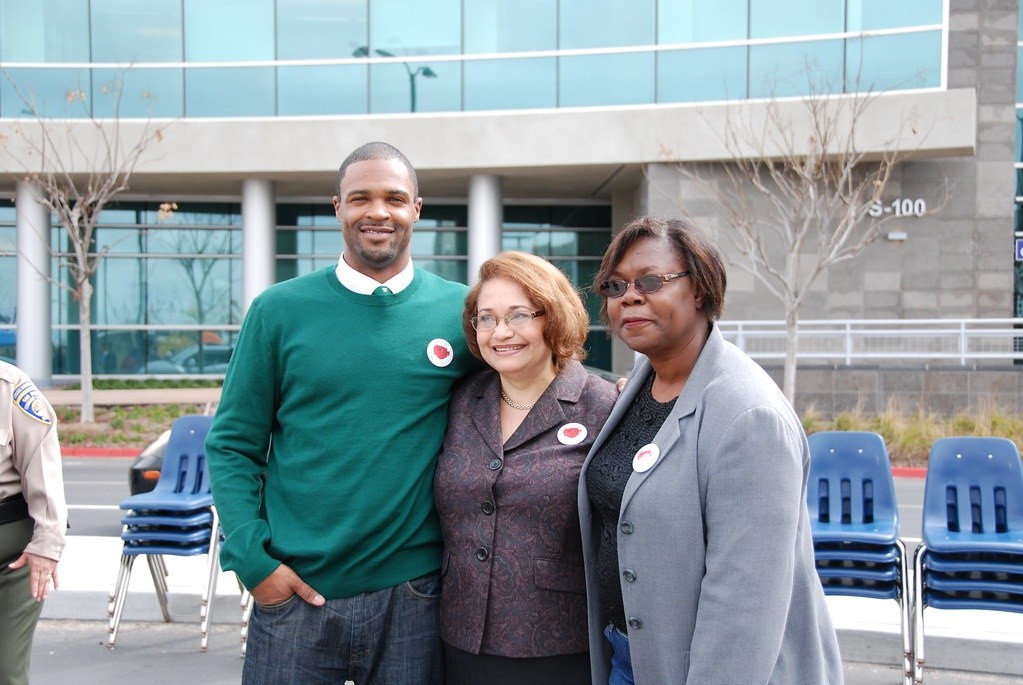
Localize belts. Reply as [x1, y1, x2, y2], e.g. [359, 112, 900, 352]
[0, 498, 29, 525]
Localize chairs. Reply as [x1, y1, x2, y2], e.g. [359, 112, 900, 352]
[807, 431, 1023, 685]
[108, 413, 253, 661]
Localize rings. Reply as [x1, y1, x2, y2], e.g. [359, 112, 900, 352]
[46, 574, 52, 578]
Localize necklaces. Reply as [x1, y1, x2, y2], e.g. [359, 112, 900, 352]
[500, 391, 535, 410]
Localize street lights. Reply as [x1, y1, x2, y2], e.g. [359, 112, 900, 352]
[354, 47, 436, 113]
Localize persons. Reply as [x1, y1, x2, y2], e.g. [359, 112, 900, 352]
[203, 143, 627, 685]
[0, 362, 70, 685]
[99, 343, 136, 373]
[573, 214, 849, 685]
[433, 249, 621, 685]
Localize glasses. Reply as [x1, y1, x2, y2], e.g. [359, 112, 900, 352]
[469, 309, 545, 332]
[599, 271, 691, 298]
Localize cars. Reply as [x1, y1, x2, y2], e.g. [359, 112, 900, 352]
[132, 345, 233, 375]
